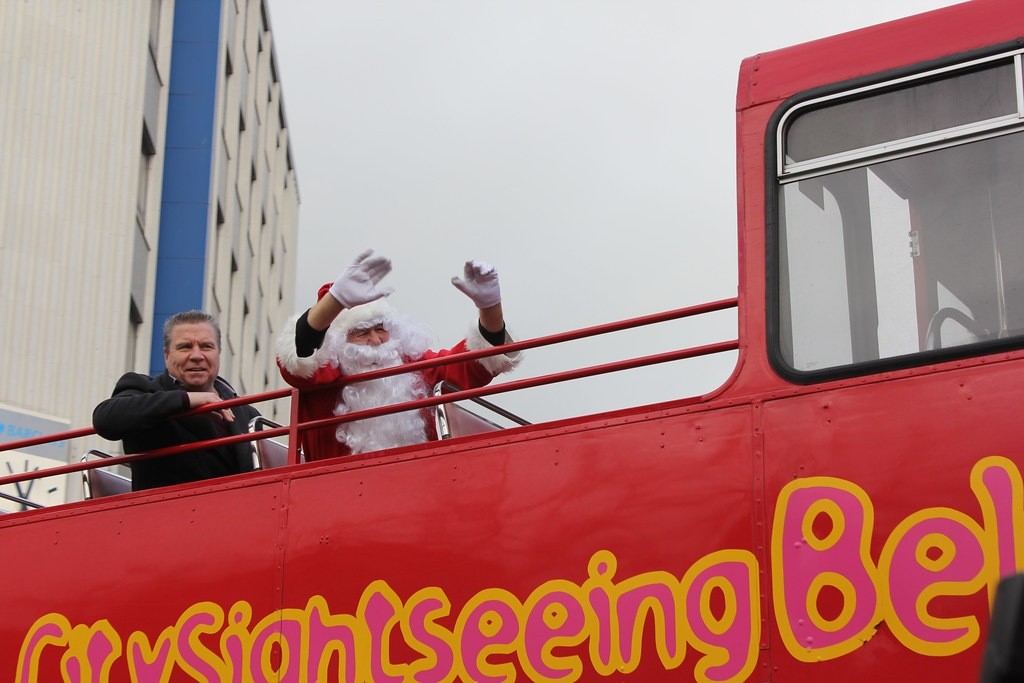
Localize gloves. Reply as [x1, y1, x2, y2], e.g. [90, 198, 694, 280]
[451, 258, 502, 309]
[329, 247, 397, 310]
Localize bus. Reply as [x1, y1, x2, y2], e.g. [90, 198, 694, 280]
[1, 0, 1024, 681]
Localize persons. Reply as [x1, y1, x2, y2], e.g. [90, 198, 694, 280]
[272, 250, 520, 463]
[91, 309, 265, 492]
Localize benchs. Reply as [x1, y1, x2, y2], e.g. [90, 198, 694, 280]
[433, 380, 539, 442]
[248, 411, 308, 469]
[78, 448, 134, 501]
